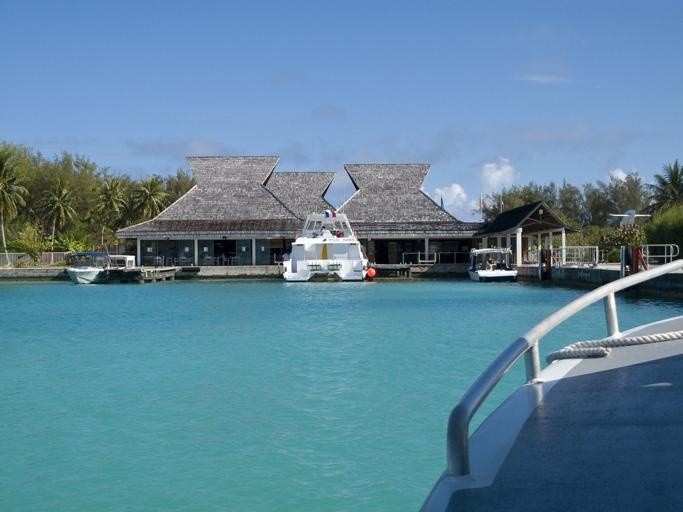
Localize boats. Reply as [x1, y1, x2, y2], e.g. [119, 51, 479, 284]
[282, 214, 368, 281]
[419, 260, 683, 511]
[468, 247, 518, 282]
[66, 252, 108, 284]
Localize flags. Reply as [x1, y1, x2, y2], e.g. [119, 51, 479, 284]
[324, 210, 336, 218]
[439, 195, 445, 209]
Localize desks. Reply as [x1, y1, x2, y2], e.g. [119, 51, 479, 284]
[107, 255, 136, 268]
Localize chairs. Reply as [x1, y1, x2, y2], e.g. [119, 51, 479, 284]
[153, 255, 240, 266]
